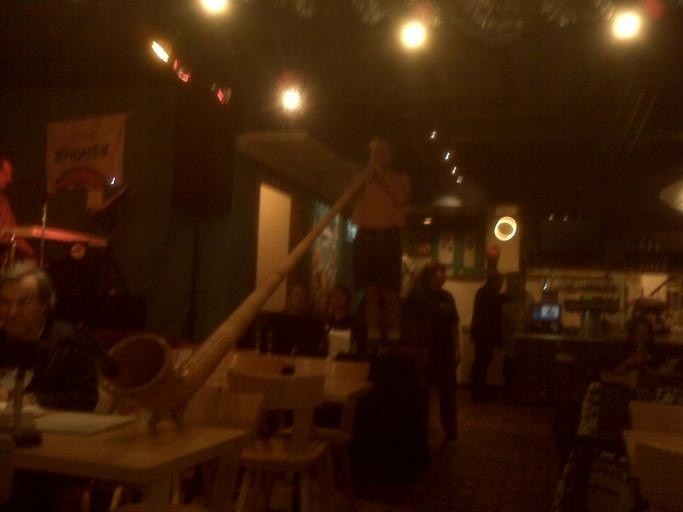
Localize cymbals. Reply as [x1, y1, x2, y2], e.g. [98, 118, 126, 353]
[0, 225, 107, 249]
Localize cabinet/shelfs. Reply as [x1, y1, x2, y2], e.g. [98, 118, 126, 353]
[505, 328, 675, 405]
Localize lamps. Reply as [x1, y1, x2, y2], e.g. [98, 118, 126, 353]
[544, 207, 574, 225]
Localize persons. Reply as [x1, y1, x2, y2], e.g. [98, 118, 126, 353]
[0, 260, 116, 512]
[303, 287, 367, 360]
[468, 268, 507, 397]
[395, 259, 462, 444]
[498, 270, 535, 336]
[0, 157, 34, 264]
[608, 308, 666, 373]
[345, 134, 406, 347]
[262, 279, 325, 362]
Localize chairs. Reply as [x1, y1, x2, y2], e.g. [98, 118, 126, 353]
[624, 398, 682, 511]
[0, 343, 374, 512]
